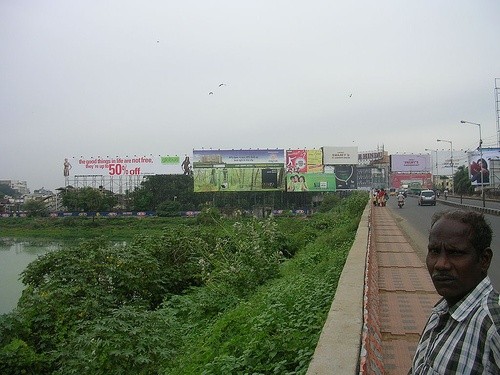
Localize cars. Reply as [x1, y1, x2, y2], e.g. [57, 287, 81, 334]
[417, 190, 437, 206]
[397, 190, 407, 198]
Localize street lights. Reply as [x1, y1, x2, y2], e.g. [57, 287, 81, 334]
[437, 139, 455, 199]
[425, 148, 439, 198]
[460, 120, 485, 207]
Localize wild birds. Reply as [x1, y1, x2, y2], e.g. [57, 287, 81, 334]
[219, 83, 223, 87]
[349, 94, 352, 98]
[209, 92, 213, 95]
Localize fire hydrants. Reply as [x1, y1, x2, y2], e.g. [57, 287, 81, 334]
[444, 190, 449, 200]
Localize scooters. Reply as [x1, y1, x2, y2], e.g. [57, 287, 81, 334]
[397, 198, 404, 208]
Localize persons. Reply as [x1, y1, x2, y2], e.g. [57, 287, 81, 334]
[471, 158, 489, 183]
[288, 175, 308, 190]
[373, 186, 449, 209]
[407, 210, 500, 375]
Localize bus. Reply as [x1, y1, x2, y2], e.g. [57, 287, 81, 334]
[389, 187, 396, 196]
